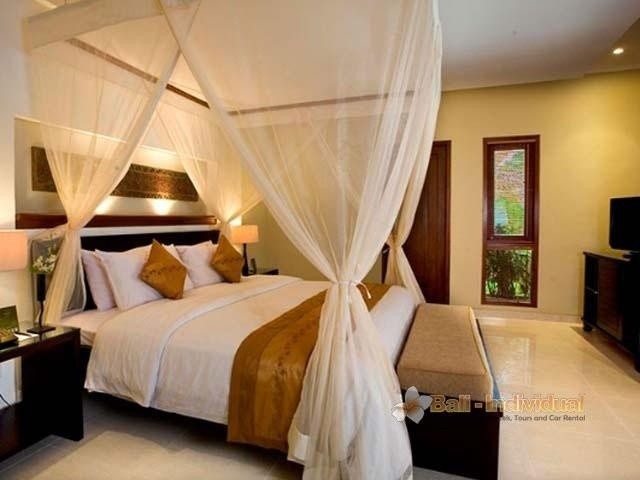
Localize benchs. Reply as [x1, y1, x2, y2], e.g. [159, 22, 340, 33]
[395, 301, 504, 480]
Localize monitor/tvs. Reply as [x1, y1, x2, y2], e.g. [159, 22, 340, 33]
[607, 195, 640, 258]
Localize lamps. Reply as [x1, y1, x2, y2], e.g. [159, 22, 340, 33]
[232, 224, 259, 276]
[0, 231, 28, 271]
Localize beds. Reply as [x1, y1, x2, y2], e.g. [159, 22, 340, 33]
[15, 213, 417, 453]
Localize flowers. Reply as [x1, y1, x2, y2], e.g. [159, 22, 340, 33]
[30, 254, 57, 274]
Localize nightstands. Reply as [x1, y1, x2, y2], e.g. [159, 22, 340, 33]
[256, 266, 279, 274]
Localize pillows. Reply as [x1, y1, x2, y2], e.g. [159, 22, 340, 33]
[95, 249, 194, 310]
[79, 249, 116, 310]
[176, 240, 222, 287]
[210, 235, 245, 283]
[137, 239, 187, 300]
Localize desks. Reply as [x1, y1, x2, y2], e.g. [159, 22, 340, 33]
[582, 250, 640, 372]
[0, 320, 83, 463]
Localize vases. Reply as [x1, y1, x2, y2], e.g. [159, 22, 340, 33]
[28, 274, 55, 333]
